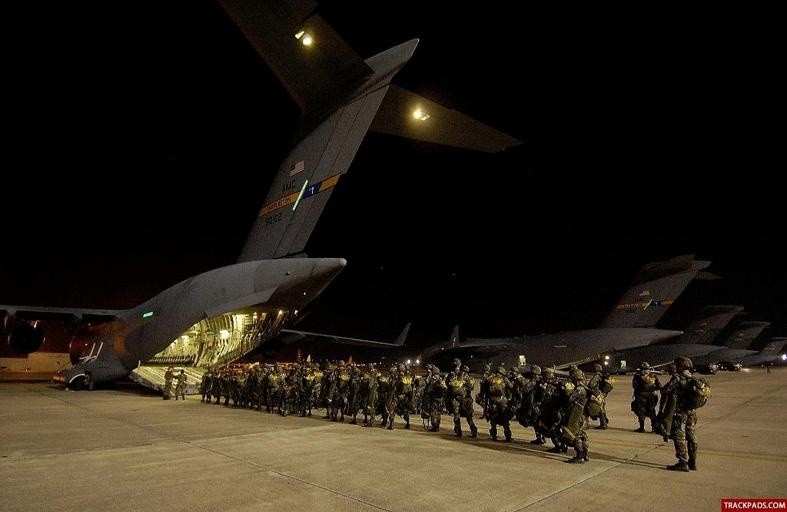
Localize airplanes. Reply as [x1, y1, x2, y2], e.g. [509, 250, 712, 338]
[0, 37, 523, 396]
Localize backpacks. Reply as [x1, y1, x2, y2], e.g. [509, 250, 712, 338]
[338, 371, 467, 400]
[577, 384, 602, 419]
[235, 371, 324, 392]
[681, 374, 711, 409]
[553, 380, 575, 398]
[488, 376, 505, 401]
[602, 375, 615, 394]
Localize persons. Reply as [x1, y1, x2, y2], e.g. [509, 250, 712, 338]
[162, 353, 713, 472]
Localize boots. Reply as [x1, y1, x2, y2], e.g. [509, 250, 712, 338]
[490, 435, 512, 441]
[668, 460, 696, 472]
[596, 424, 609, 429]
[429, 425, 439, 432]
[634, 427, 645, 432]
[530, 436, 590, 463]
[201, 398, 312, 418]
[456, 433, 477, 438]
[322, 414, 410, 430]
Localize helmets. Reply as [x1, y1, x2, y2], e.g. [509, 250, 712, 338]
[593, 364, 603, 371]
[338, 360, 439, 374]
[667, 355, 693, 376]
[569, 365, 584, 380]
[453, 358, 469, 371]
[483, 364, 554, 380]
[208, 361, 319, 376]
[641, 362, 649, 370]
